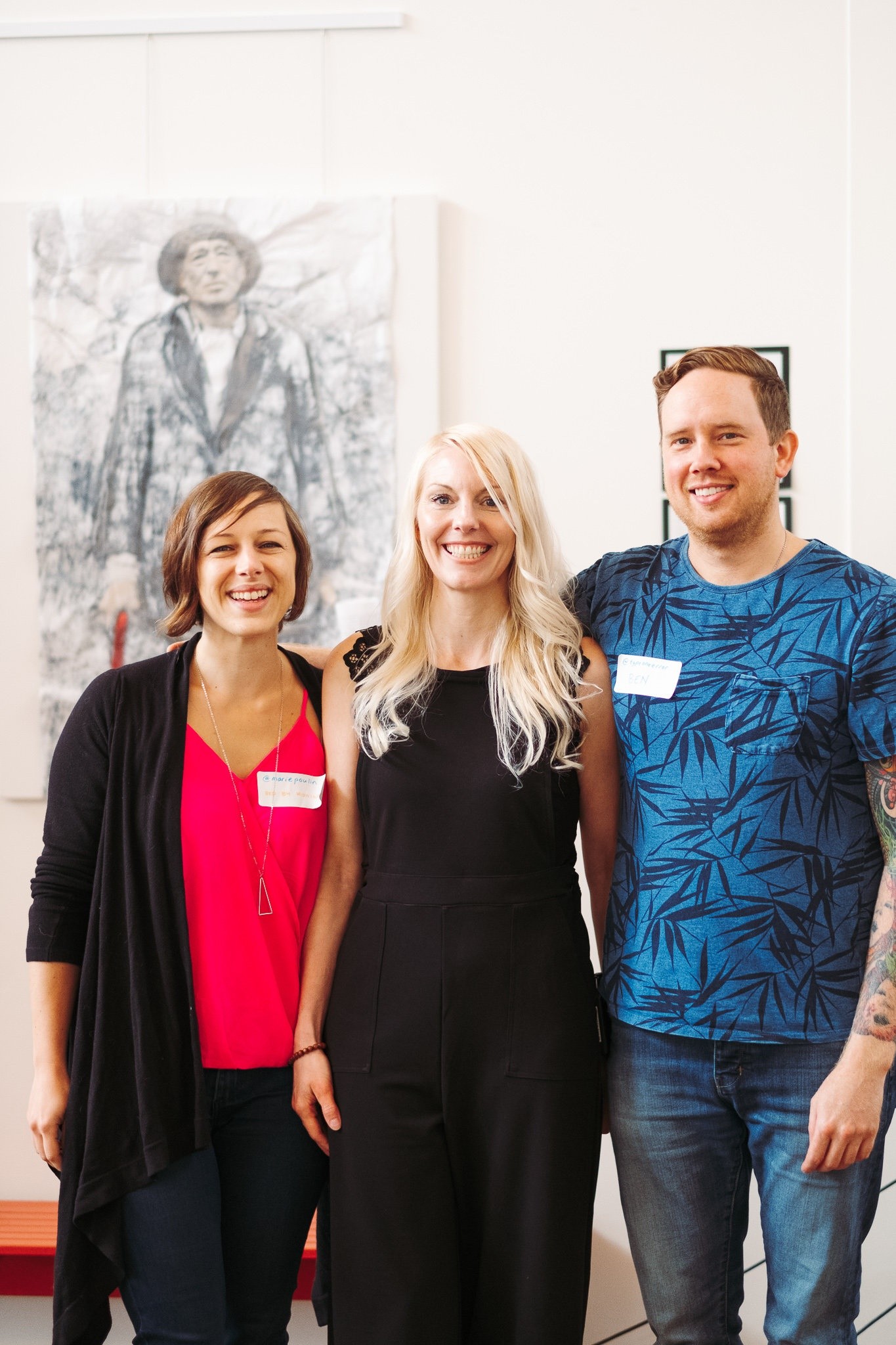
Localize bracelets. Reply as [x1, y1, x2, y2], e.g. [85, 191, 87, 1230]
[288, 1042, 327, 1066]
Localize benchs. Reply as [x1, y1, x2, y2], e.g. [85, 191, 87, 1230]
[0, 1200, 317, 1301]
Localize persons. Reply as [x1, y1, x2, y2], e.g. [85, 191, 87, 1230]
[286, 413, 620, 1345]
[19, 467, 348, 1345]
[164, 347, 895, 1345]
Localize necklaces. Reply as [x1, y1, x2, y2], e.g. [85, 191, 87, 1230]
[191, 647, 284, 921]
